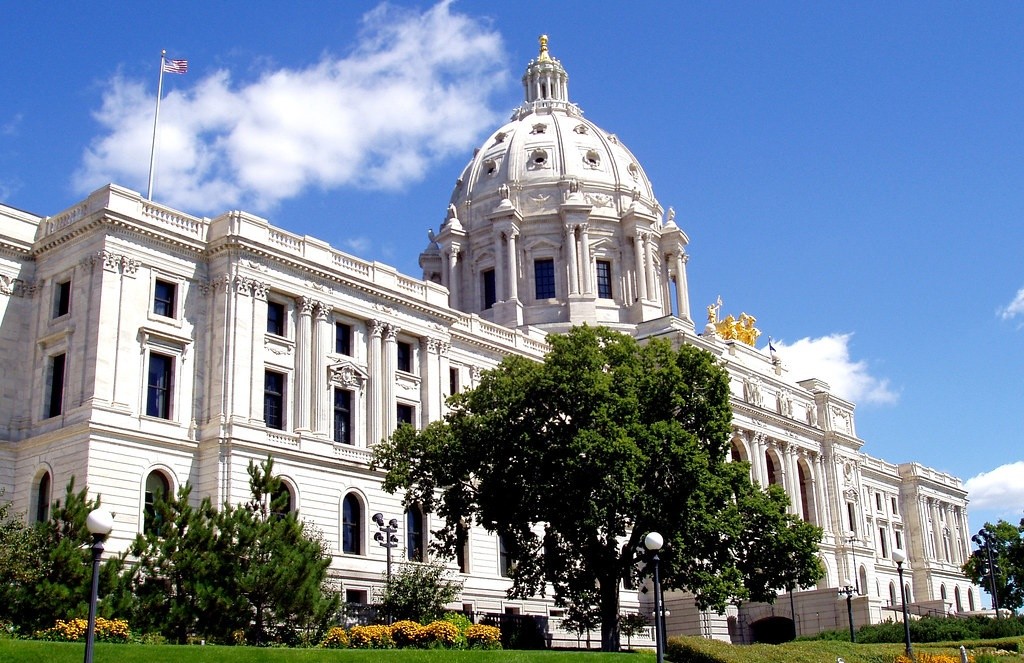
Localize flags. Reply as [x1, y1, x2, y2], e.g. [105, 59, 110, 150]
[163, 58, 188, 74]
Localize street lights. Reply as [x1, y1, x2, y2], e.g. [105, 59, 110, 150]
[370, 512, 400, 624]
[846, 531, 861, 597]
[971, 528, 999, 620]
[842, 578, 856, 642]
[84, 509, 114, 662]
[645, 532, 665, 663]
[892, 548, 914, 655]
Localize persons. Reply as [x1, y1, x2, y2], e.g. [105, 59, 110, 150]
[707, 304, 718, 322]
